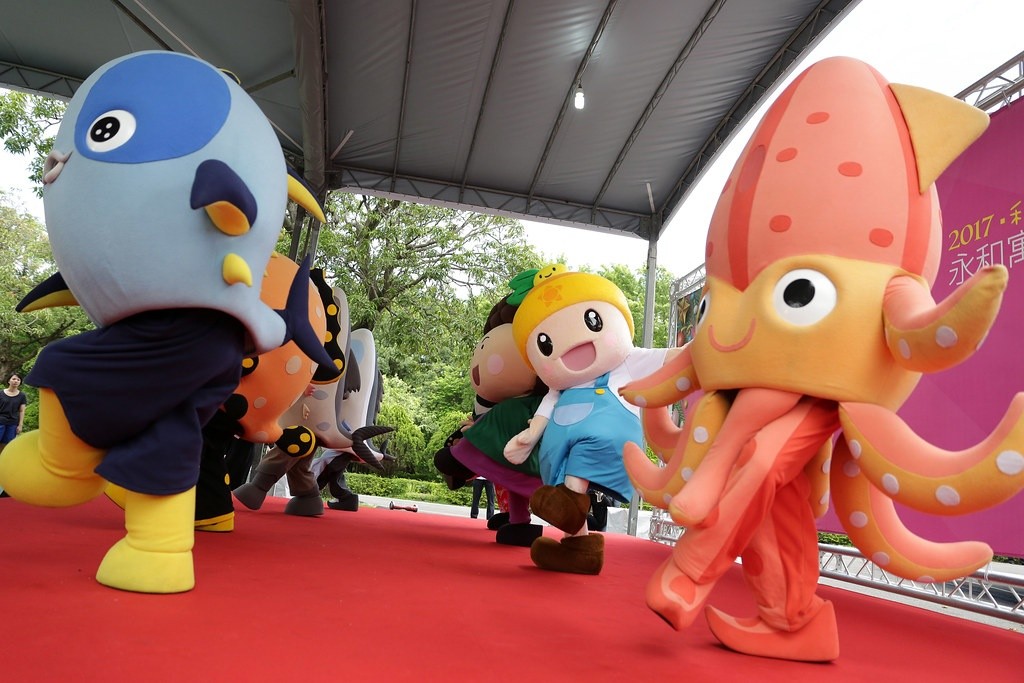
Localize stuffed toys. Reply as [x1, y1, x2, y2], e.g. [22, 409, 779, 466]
[433, 292, 546, 547]
[230, 287, 395, 516]
[620, 55, 1024, 661]
[0, 49, 325, 595]
[504, 264, 697, 574]
[101, 249, 347, 534]
[307, 328, 397, 511]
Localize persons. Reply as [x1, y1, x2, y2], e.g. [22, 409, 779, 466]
[0, 372, 26, 455]
[469, 475, 496, 520]
